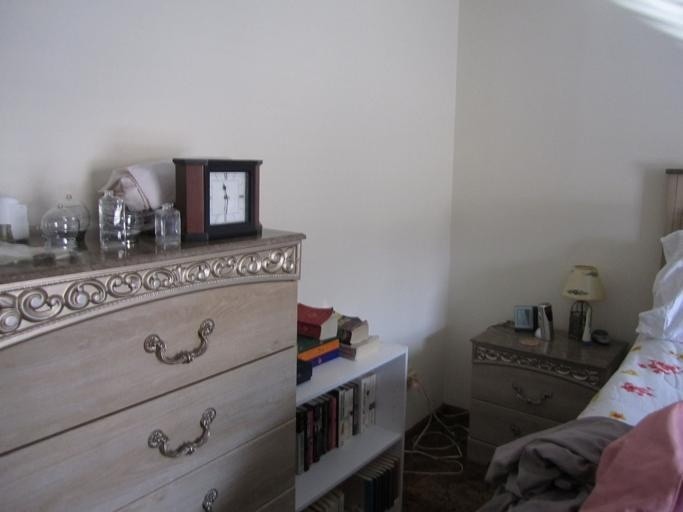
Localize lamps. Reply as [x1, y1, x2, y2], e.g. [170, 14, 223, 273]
[561, 265, 604, 342]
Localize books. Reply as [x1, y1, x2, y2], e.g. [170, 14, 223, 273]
[297, 300, 402, 512]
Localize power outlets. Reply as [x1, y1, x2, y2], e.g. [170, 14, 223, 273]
[407, 370, 420, 389]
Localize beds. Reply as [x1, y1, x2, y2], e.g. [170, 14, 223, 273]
[478, 169, 683, 511]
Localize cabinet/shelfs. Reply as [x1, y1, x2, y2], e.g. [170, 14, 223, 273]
[295, 337, 409, 512]
[0, 227, 306, 512]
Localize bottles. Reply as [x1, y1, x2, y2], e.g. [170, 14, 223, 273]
[154, 202, 182, 247]
[99, 189, 128, 247]
[126, 211, 144, 243]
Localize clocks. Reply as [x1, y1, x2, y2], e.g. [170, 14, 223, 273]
[172, 159, 263, 241]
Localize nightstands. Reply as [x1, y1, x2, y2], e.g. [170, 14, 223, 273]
[464, 321, 629, 491]
